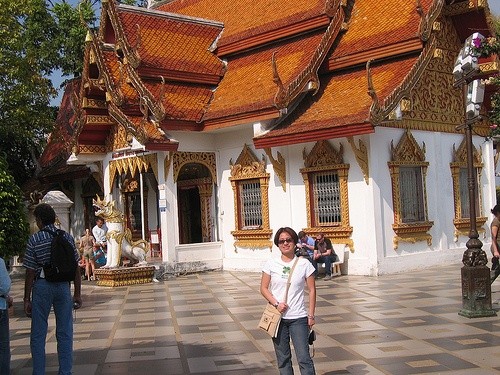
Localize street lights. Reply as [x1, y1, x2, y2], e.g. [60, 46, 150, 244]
[452, 33, 497, 318]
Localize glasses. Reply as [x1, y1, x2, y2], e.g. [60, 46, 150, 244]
[315, 237, 323, 241]
[278, 238, 295, 244]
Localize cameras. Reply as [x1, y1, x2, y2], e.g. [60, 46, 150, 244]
[309, 330, 316, 345]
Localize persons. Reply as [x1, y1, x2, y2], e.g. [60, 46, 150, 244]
[0, 258, 13, 375]
[295, 231, 315, 259]
[80, 229, 106, 281]
[312, 234, 337, 280]
[260, 227, 316, 375]
[92, 218, 108, 243]
[22, 203, 83, 375]
[490, 205, 500, 285]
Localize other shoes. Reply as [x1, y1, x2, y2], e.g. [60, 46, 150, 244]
[322, 272, 331, 281]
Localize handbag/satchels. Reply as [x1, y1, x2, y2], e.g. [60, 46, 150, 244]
[294, 246, 310, 258]
[258, 302, 283, 338]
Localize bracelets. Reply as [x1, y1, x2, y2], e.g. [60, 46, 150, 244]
[308, 315, 315, 319]
[321, 253, 322, 255]
[273, 302, 279, 307]
[23, 297, 29, 301]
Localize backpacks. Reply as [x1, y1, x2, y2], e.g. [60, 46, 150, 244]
[40, 228, 77, 282]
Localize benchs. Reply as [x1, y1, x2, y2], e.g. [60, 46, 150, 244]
[313, 244, 347, 277]
